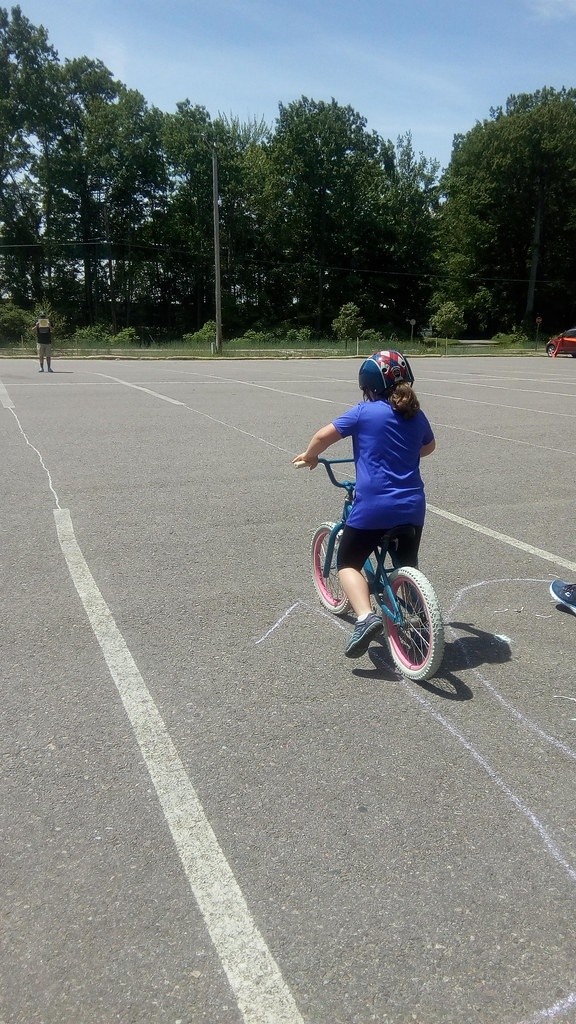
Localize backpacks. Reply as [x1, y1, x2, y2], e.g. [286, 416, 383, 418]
[36, 319, 51, 334]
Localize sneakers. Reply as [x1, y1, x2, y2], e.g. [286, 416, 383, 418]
[345, 606, 384, 659]
[550, 579, 576, 614]
[410, 627, 429, 645]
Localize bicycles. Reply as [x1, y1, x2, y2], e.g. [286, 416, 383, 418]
[294, 458, 445, 681]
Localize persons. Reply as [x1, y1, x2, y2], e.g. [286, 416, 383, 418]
[32, 314, 54, 372]
[549, 579, 576, 614]
[292, 351, 435, 658]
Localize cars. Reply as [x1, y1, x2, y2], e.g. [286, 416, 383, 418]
[545, 328, 576, 358]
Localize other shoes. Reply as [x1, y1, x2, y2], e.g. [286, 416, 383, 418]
[38, 368, 44, 372]
[48, 368, 53, 372]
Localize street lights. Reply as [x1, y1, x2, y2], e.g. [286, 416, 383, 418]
[193, 132, 222, 357]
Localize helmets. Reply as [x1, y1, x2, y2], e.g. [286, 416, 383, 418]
[359, 349, 415, 395]
[39, 311, 47, 316]
